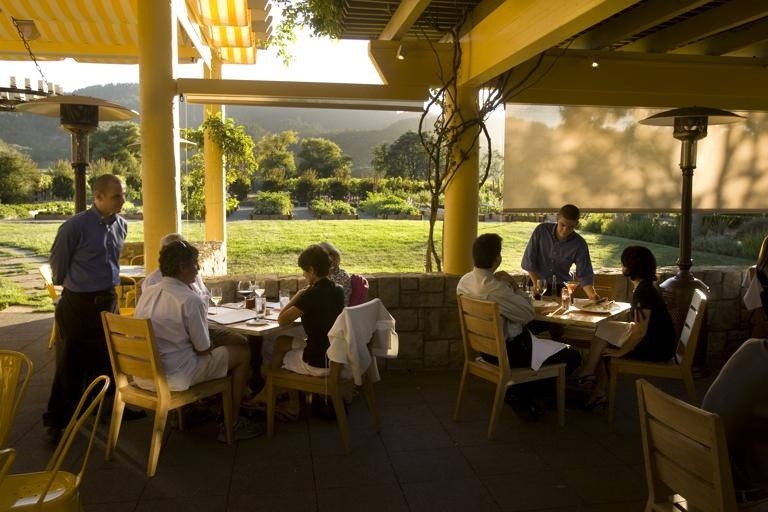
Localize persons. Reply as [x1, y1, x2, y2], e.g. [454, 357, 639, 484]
[316, 240, 352, 306]
[243, 247, 345, 423]
[41, 173, 128, 442]
[128, 242, 265, 444]
[519, 203, 602, 339]
[454, 232, 579, 417]
[754, 237, 768, 320]
[139, 233, 254, 394]
[567, 246, 675, 414]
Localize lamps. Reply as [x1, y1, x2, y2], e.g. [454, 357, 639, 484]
[0, 15, 138, 219]
[641, 103, 748, 304]
[396, 44, 408, 60]
[589, 56, 600, 69]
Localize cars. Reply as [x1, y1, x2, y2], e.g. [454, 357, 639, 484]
[721, 216, 754, 239]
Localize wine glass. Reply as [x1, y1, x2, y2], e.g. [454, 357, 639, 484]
[206, 279, 301, 325]
[517, 276, 579, 315]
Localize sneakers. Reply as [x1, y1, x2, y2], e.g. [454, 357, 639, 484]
[44, 426, 62, 443]
[505, 391, 545, 421]
[123, 407, 147, 420]
[218, 417, 263, 442]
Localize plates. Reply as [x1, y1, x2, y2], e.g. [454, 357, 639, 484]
[572, 299, 621, 314]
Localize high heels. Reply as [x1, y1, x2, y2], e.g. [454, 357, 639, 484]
[579, 391, 608, 411]
[567, 370, 596, 391]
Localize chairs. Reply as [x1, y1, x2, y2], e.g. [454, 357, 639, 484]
[633, 374, 767, 511]
[0, 347, 114, 512]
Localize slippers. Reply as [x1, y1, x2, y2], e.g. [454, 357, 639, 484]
[241, 397, 299, 422]
